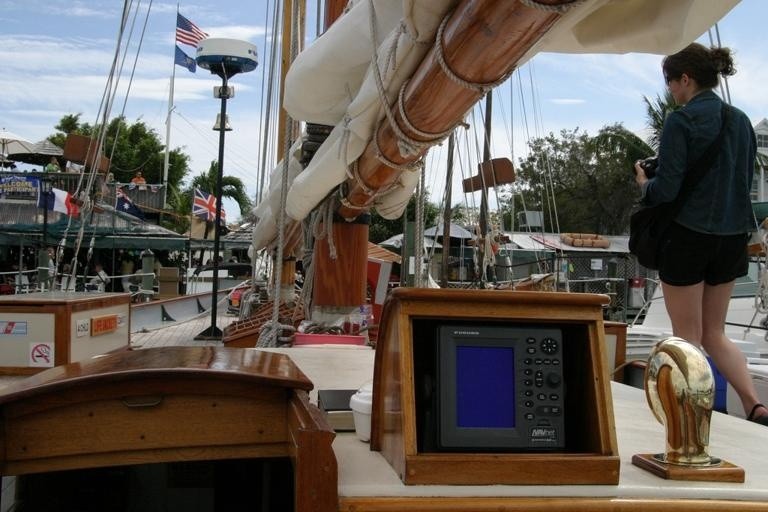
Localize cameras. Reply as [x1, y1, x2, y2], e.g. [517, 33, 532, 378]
[633, 153, 658, 178]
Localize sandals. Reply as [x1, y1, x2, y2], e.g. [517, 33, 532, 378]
[746, 403, 768, 426]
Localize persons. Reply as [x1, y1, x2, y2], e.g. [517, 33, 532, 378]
[90, 263, 111, 292]
[131, 172, 146, 184]
[48, 247, 55, 276]
[107, 172, 120, 183]
[634, 43, 768, 424]
[47, 157, 60, 172]
[118, 256, 133, 293]
[66, 160, 84, 173]
[8, 265, 30, 284]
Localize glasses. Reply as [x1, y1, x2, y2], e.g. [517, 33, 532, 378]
[665, 75, 682, 86]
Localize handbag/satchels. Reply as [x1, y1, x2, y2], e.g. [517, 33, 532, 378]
[628, 206, 676, 269]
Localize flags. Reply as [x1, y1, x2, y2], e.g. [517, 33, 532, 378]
[37, 180, 79, 218]
[115, 188, 146, 221]
[192, 188, 225, 224]
[174, 13, 209, 74]
